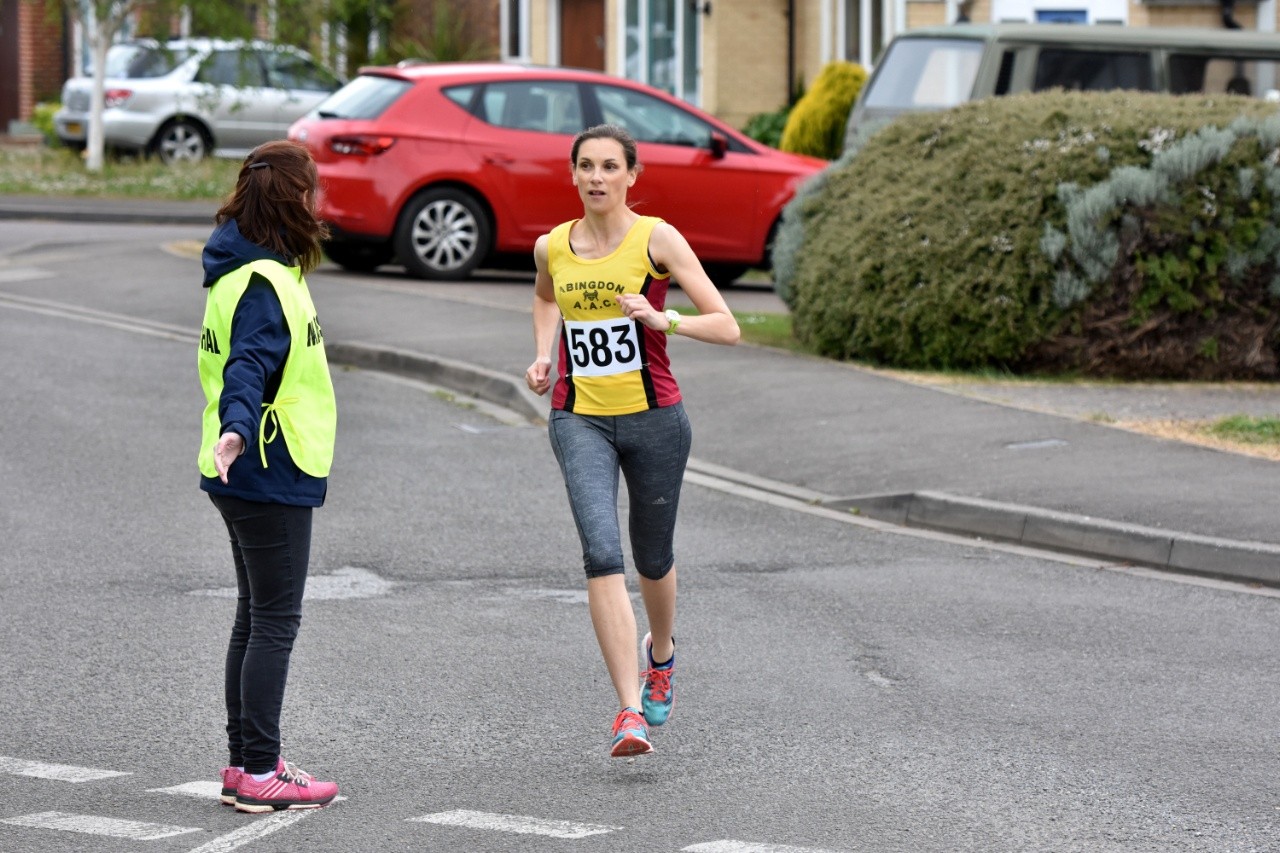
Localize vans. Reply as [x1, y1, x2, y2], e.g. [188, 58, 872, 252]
[842, 20, 1280, 160]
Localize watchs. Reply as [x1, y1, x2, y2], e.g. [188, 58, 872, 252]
[667, 309, 681, 335]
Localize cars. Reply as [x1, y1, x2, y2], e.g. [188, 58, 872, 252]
[287, 57, 835, 289]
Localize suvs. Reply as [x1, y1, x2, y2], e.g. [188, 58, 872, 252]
[56, 34, 343, 168]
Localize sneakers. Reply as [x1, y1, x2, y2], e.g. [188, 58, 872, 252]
[234, 756, 338, 812]
[220, 755, 312, 806]
[610, 707, 654, 757]
[640, 632, 676, 726]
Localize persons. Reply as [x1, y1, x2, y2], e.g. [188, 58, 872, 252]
[198, 141, 339, 812]
[525, 123, 739, 757]
